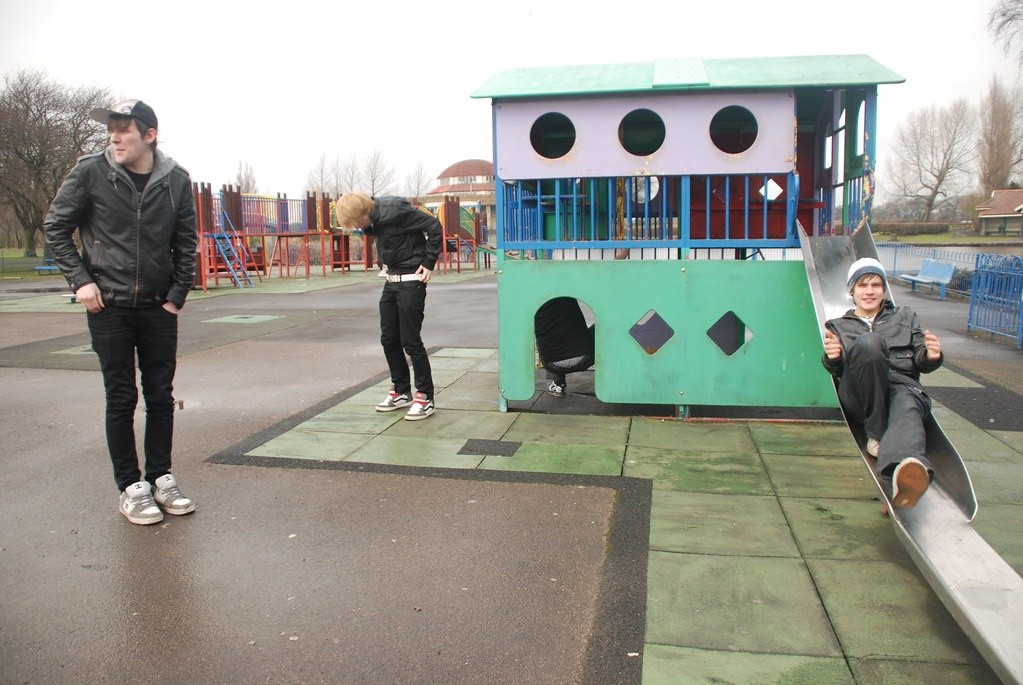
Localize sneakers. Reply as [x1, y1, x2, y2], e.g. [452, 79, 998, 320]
[118, 481, 164, 524]
[545, 380, 568, 397]
[375, 387, 414, 412]
[404, 391, 435, 421]
[151, 474, 195, 515]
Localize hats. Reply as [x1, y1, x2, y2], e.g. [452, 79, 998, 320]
[846, 257, 887, 295]
[91, 99, 158, 132]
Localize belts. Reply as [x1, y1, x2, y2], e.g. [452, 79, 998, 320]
[387, 272, 423, 283]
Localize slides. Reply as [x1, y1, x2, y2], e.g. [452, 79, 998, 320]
[794, 215, 1022, 684]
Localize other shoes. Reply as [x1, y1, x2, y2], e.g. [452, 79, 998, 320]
[867, 438, 881, 457]
[892, 457, 929, 510]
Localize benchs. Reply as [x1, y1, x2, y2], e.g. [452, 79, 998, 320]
[33, 266, 60, 270]
[901, 257, 958, 300]
[674, 197, 825, 238]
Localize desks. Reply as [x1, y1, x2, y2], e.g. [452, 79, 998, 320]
[44, 258, 55, 275]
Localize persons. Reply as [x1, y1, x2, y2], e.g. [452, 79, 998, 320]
[533, 295, 595, 398]
[41, 98, 196, 525]
[820, 255, 944, 510]
[334, 190, 444, 422]
